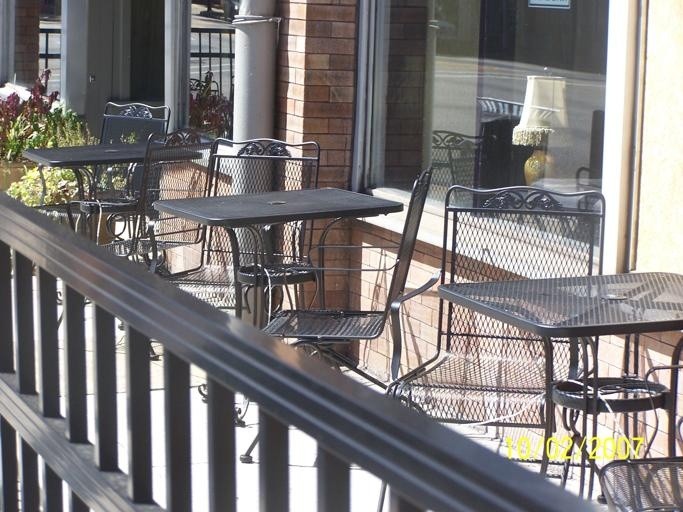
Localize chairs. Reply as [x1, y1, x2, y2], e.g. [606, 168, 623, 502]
[383, 184, 683, 511]
[431, 100, 561, 212]
[22, 101, 226, 303]
[152, 139, 433, 464]
[24, 100, 682, 512]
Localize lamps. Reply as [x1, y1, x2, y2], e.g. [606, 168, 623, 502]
[509, 74, 574, 186]
